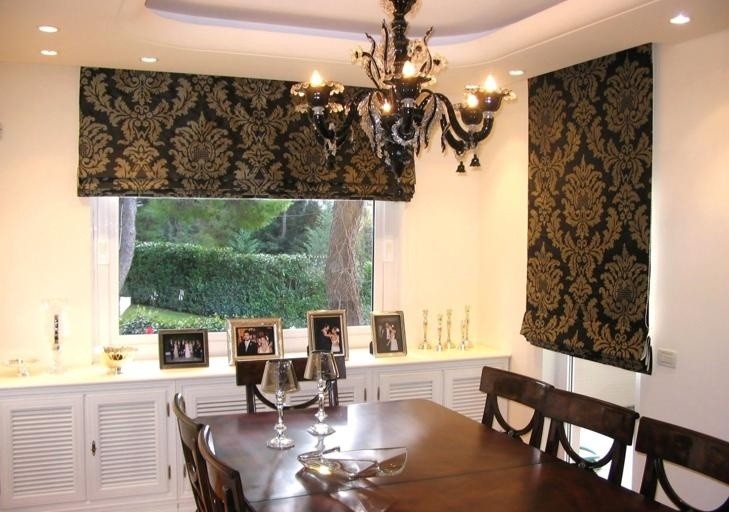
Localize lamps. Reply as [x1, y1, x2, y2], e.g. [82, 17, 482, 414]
[290, 1, 517, 182]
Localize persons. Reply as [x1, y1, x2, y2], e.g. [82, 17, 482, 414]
[236, 325, 277, 355]
[320, 325, 340, 352]
[317, 321, 333, 350]
[164, 335, 205, 359]
[380, 320, 397, 351]
[386, 323, 398, 351]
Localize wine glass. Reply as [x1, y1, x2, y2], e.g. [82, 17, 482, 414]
[304, 350, 339, 436]
[260, 360, 299, 449]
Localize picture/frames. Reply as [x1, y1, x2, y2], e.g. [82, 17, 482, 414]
[158, 310, 408, 370]
